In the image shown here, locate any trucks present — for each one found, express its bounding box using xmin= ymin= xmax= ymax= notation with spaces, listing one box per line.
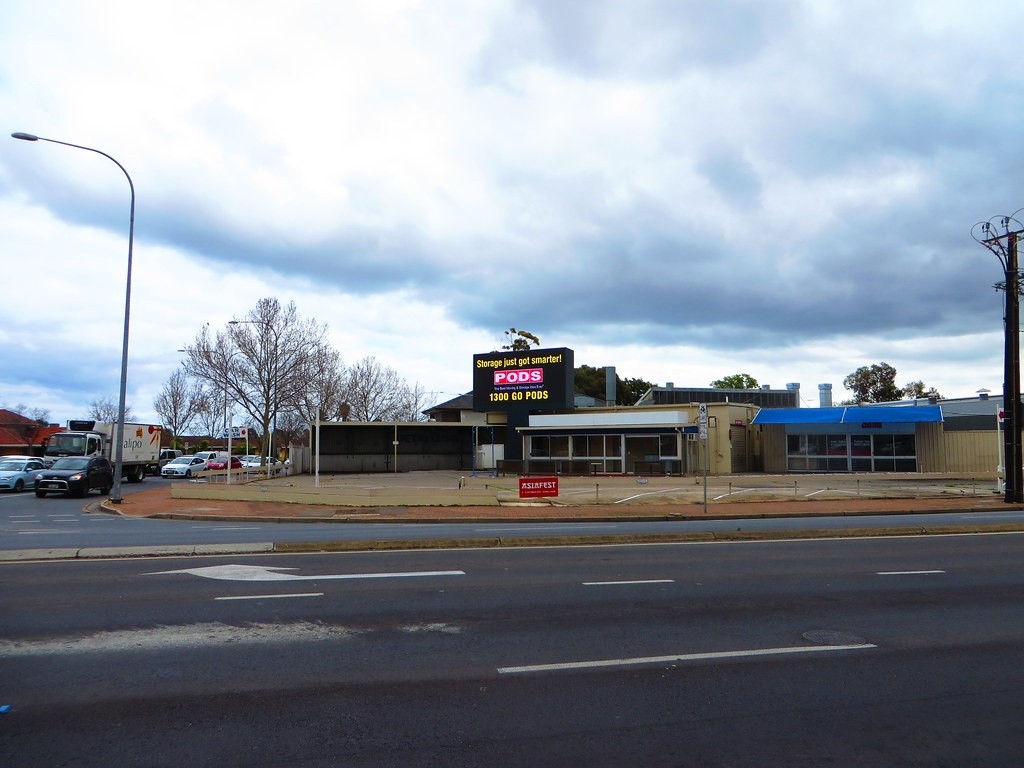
xmin=193 ymin=451 xmax=229 ymax=464
xmin=41 ymin=419 xmax=163 ymax=483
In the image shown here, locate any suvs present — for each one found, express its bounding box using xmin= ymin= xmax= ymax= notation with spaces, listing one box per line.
xmin=145 ymin=449 xmax=184 ymax=477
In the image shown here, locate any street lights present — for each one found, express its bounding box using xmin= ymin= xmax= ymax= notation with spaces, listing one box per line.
xmin=11 ymin=132 xmax=135 ymax=504
xmin=177 ymin=349 xmax=227 ymax=452
xmin=228 ymin=320 xmax=278 ymax=474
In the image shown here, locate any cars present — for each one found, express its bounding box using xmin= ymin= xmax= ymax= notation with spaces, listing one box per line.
xmin=161 ymin=456 xmax=208 ymax=478
xmin=207 ymin=456 xmax=242 ymax=475
xmin=242 ymin=456 xmax=283 ymax=476
xmin=34 ymin=456 xmax=115 ymax=500
xmin=0 ymin=461 xmax=51 ymax=494
xmin=238 ymin=455 xmax=258 ymax=466
xmin=0 ymin=455 xmax=44 ymax=467
